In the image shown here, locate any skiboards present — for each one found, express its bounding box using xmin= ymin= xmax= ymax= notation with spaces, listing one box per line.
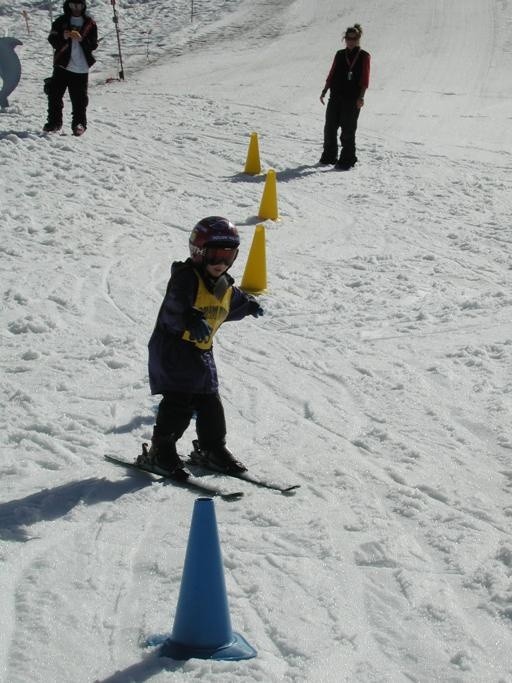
xmin=105 ymin=452 xmax=301 ymax=497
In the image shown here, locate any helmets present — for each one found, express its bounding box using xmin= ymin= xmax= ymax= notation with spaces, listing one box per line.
xmin=189 ymin=215 xmax=239 ymax=268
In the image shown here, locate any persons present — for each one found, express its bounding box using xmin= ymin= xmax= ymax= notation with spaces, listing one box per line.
xmin=142 ymin=216 xmax=265 ymax=478
xmin=319 ymin=24 xmax=371 ymax=170
xmin=43 ymin=0 xmax=98 ymax=137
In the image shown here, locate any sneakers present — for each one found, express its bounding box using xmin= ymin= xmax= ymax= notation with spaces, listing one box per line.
xmin=200 ymin=447 xmax=248 ymax=473
xmin=73 ymin=124 xmax=85 ymax=135
xmin=43 ymin=123 xmax=59 ymax=129
xmin=148 ymin=445 xmax=185 ymax=473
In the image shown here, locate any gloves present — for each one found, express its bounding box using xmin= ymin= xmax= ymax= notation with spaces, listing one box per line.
xmin=183 ymin=309 xmax=212 ymax=341
xmin=246 ymin=299 xmax=264 ymax=318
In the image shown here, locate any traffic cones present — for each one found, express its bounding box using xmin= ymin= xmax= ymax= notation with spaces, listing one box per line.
xmin=243 ymin=131 xmax=265 ymax=177
xmin=166 ymin=494 xmax=257 ymax=662
xmin=239 ymin=224 xmax=268 ymax=295
xmin=260 ymin=169 xmax=282 ymax=221
